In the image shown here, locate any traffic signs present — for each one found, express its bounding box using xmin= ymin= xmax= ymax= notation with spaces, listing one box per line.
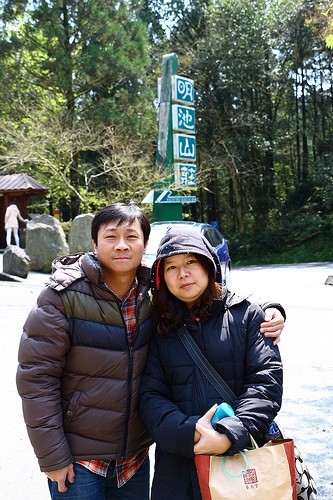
xmin=155 ymin=191 xmax=197 ymax=204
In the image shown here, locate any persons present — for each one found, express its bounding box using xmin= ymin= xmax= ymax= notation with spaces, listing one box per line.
xmin=16 ymin=202 xmax=286 ymax=500
xmin=4 ymin=200 xmax=27 ymax=246
xmin=141 ymin=226 xmax=283 ymax=500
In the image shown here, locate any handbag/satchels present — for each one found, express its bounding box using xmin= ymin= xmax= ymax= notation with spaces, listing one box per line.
xmin=291 ymin=446 xmax=317 ymax=500
xmin=194 ymin=434 xmax=298 ymax=500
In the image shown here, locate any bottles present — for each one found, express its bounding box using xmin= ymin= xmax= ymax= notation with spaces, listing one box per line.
xmin=211 ymin=402 xmax=235 ymax=429
